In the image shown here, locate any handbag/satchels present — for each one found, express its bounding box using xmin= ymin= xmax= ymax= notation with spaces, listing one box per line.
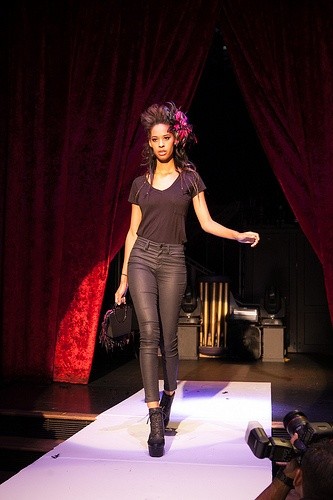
xmin=104 ymin=303 xmax=132 ymax=339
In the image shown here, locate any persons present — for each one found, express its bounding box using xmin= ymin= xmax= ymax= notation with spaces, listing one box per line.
xmin=115 ymin=104 xmax=260 ymax=456
xmin=255 ymin=432 xmax=333 ymax=500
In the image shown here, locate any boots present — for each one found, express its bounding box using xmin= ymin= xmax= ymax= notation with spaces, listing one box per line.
xmin=142 ymin=407 xmax=165 ymax=456
xmin=159 ymin=391 xmax=174 ymax=429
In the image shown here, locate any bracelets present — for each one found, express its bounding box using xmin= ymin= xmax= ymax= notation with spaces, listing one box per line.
xmin=122 ymin=274 xmax=127 ymax=276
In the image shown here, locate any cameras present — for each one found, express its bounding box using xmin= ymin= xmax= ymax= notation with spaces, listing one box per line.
xmin=244 ymin=409 xmax=333 ymax=466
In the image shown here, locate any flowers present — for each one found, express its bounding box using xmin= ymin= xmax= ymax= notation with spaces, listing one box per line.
xmin=174 ymin=108 xmax=198 ymax=146
